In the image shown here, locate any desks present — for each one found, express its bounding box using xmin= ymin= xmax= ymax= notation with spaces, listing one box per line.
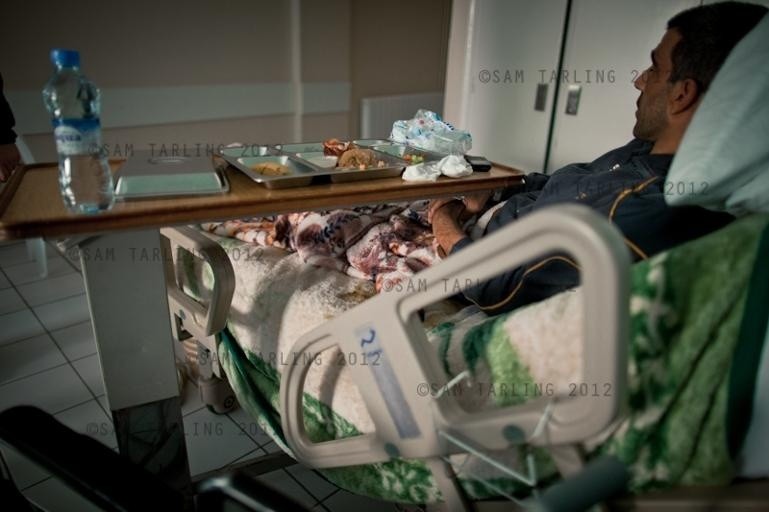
xmin=1 ymin=143 xmax=531 ymax=484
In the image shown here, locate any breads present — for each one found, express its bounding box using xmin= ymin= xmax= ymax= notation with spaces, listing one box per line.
xmin=338 ymin=149 xmax=385 ymax=170
xmin=323 ymin=138 xmax=361 ymax=156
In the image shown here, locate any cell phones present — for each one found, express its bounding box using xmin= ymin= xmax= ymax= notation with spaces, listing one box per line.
xmin=466 ymin=156 xmax=491 ymax=172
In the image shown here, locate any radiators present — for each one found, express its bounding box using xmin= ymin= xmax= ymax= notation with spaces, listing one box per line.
xmin=360 ymin=92 xmax=444 ymax=140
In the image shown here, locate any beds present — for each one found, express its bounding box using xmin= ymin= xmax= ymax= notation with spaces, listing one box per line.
xmin=159 ymin=176 xmax=769 ymax=512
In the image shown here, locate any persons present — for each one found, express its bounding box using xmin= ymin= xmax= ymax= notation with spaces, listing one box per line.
xmin=426 ymin=1 xmax=769 ymax=321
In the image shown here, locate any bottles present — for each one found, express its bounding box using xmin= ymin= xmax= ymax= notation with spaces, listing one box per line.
xmin=41 ymin=49 xmax=114 ymax=215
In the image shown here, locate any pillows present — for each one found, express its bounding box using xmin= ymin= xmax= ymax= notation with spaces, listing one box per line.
xmin=663 ymin=15 xmax=769 ymax=218
